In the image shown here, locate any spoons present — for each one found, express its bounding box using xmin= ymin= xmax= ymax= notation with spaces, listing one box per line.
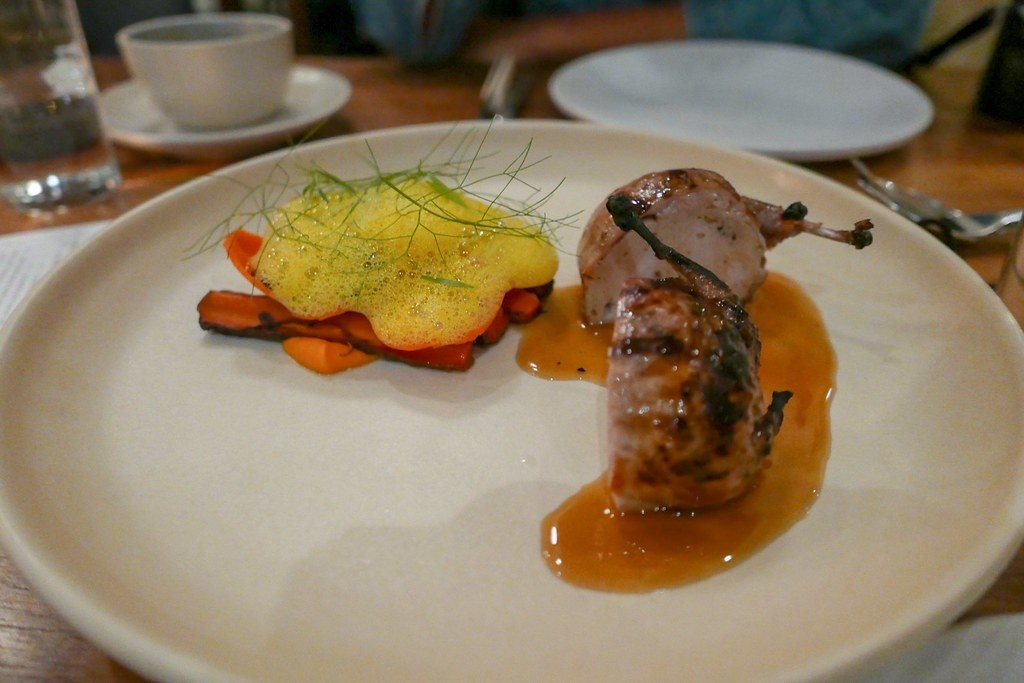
xmin=853 ymin=160 xmax=1024 ymax=243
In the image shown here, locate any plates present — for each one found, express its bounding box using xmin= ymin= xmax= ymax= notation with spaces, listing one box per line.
xmin=103 ymin=65 xmax=353 ymax=161
xmin=0 ymin=120 xmax=1024 ymax=683
xmin=549 ymin=41 xmax=936 ymax=162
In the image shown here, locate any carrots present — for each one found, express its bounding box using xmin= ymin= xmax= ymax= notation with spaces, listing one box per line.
xmin=195 ymin=231 xmax=542 ymax=370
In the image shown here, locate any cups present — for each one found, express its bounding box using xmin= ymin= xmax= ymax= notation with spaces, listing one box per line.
xmin=115 ymin=12 xmax=294 ymax=130
xmin=683 ymin=0 xmax=931 ymax=70
xmin=978 ymin=0 xmax=1024 ymax=125
xmin=0 ymin=0 xmax=121 ymax=215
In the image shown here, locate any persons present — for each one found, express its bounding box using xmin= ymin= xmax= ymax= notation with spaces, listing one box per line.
xmin=353 ymin=0 xmax=935 ymax=67
